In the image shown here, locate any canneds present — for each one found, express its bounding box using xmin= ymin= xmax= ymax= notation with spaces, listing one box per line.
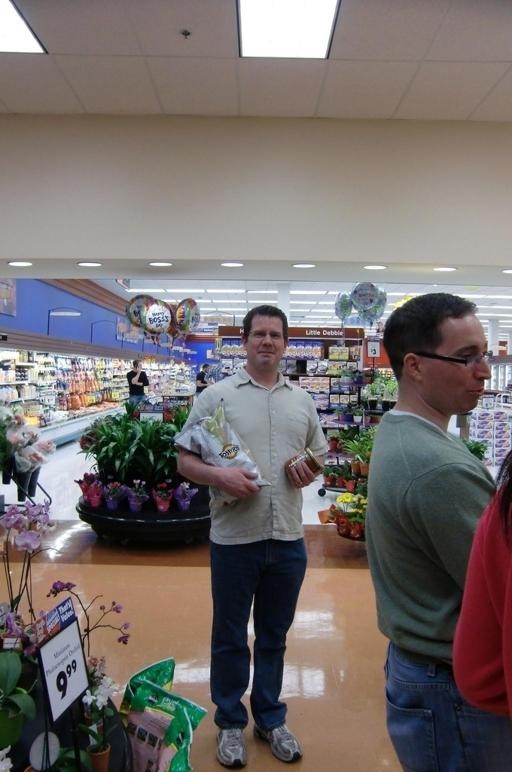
xmin=285 ymin=446 xmax=320 ymax=479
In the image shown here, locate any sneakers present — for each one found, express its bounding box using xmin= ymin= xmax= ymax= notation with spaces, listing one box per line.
xmin=254 ymin=723 xmax=303 ymax=762
xmin=216 ymin=728 xmax=246 ymax=767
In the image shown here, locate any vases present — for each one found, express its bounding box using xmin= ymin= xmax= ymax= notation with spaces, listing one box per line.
xmin=80 ymin=485 xmax=192 ymax=511
xmin=336 ymin=516 xmax=364 ymax=538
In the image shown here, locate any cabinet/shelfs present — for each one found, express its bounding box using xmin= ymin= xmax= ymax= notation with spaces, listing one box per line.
xmin=216 ymin=336 xmax=364 ymax=412
xmin=0 ymin=346 xmax=198 ymax=453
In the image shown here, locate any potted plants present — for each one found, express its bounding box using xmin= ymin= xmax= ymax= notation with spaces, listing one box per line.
xmin=80 ymin=685 xmax=111 ymax=772
xmin=322 ymin=402 xmax=379 ymax=493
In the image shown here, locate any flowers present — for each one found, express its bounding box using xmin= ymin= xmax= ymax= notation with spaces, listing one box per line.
xmin=78 ymin=474 xmax=199 ymax=500
xmin=336 ymin=492 xmax=367 ymax=523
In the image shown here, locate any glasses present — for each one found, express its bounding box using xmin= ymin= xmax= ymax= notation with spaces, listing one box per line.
xmin=414 ymin=350 xmax=493 ymax=367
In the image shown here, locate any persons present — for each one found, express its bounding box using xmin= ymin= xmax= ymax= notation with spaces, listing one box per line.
xmin=173 ymin=304 xmax=332 ymax=769
xmin=195 ymin=363 xmax=210 ymax=397
xmin=450 ymin=449 xmax=512 ymax=728
xmin=127 ymin=359 xmax=150 ymax=406
xmin=362 ymin=291 xmax=512 ymax=772
